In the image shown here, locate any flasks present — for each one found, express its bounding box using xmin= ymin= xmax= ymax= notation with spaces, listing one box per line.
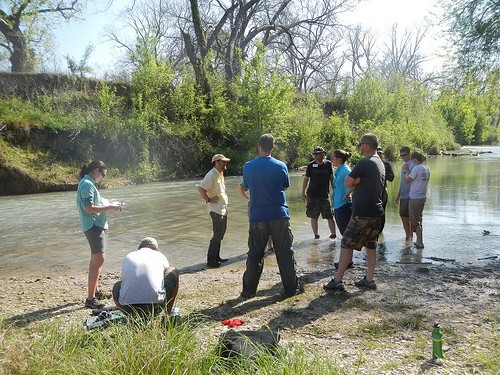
xmin=432 ymin=321 xmax=442 ymax=359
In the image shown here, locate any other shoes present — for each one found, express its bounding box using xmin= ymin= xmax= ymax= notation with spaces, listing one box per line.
xmin=244 ymin=291 xmax=254 ymax=298
xmin=218 ymin=259 xmax=228 ymax=262
xmin=315 ymin=235 xmax=319 ymax=238
xmin=330 ymin=235 xmax=336 ymax=238
xmin=208 ymin=261 xmax=221 ymax=266
xmin=334 ymin=261 xmax=353 ymax=267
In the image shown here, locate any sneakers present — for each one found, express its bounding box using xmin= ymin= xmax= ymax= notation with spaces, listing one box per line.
xmin=323 ymin=279 xmax=343 ymax=291
xmin=358 ymin=276 xmax=376 ymax=288
xmin=85 ymin=298 xmax=105 ymax=309
xmin=95 ymin=291 xmax=112 ymax=299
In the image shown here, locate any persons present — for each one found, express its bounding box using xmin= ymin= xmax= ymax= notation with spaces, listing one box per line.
xmin=395 ymin=146 xmax=413 ymax=241
xmin=241 ymin=133 xmax=305 ymax=299
xmin=238 ymin=181 xmax=274 ymax=255
xmin=323 ymin=132 xmax=386 ymax=291
xmin=403 ymin=151 xmax=430 ymax=249
xmin=376 ymin=146 xmax=394 ymax=243
xmin=76 ymin=160 xmax=124 ymax=308
xmin=302 ymin=146 xmax=336 ymax=239
xmin=198 ymin=154 xmax=230 ymax=268
xmin=332 ymin=149 xmax=354 ymax=268
xmin=113 ymin=236 xmax=181 ymax=324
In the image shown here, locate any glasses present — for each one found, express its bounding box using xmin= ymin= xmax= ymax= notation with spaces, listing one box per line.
xmin=400 ymin=153 xmax=409 ymax=157
xmin=359 ymin=142 xmax=370 ymax=146
xmin=100 ymin=171 xmax=105 ymax=178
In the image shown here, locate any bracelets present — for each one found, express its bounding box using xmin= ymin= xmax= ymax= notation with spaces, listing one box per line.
xmin=345 ymin=193 xmax=349 ymax=196
xmin=404 ymin=171 xmax=407 ymax=174
xmin=206 ymin=198 xmax=210 ymax=204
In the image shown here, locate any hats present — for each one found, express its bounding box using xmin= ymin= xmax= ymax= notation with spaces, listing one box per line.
xmin=314 ymin=148 xmax=325 ymax=153
xmin=212 ymin=154 xmax=230 ymax=162
xmin=377 ymin=146 xmax=382 ymax=153
